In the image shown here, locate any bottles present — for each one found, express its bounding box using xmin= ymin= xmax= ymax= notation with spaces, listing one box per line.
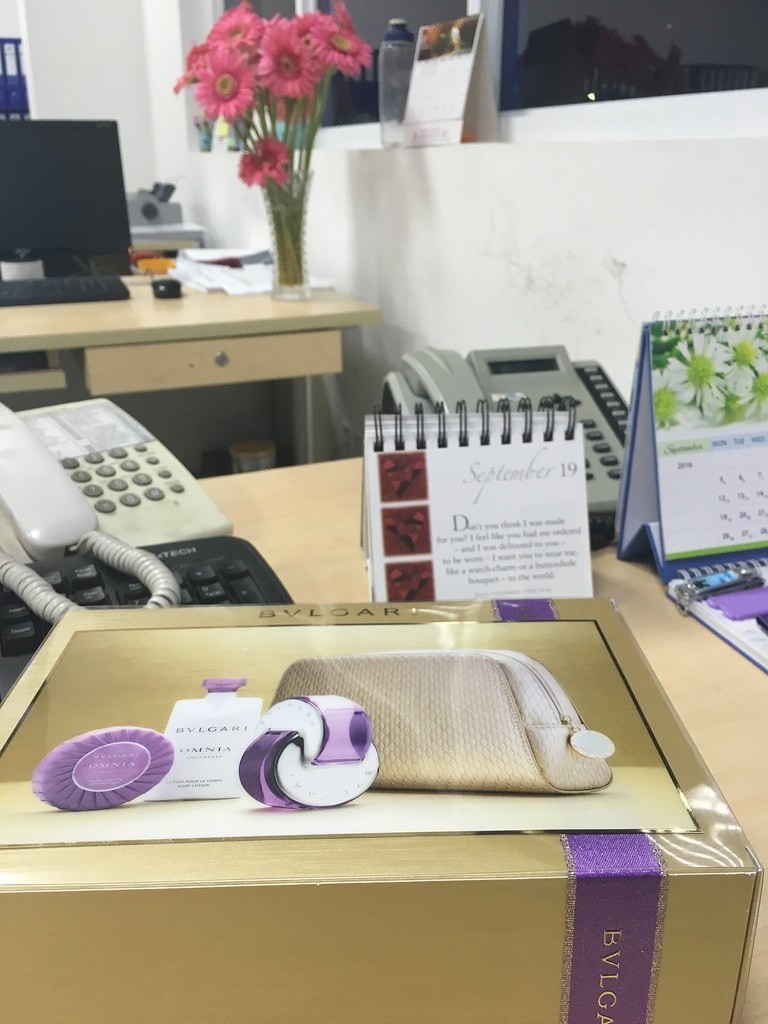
xmin=143 ymin=678 xmax=265 ymax=800
xmin=378 ymin=18 xmax=416 ymax=148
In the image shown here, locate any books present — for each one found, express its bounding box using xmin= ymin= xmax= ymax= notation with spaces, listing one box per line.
xmin=163 ymin=243 xmax=276 ymax=293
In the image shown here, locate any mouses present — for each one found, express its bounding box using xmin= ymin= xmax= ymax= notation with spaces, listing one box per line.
xmin=153 ymin=279 xmax=180 ymax=298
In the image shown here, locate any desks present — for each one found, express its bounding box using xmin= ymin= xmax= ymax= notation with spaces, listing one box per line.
xmin=0 ymin=274 xmax=384 ymax=464
xmin=192 ymin=459 xmax=768 ymax=1024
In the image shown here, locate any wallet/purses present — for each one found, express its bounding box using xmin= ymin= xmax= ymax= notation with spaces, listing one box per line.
xmin=268 ymin=650 xmax=615 ymax=793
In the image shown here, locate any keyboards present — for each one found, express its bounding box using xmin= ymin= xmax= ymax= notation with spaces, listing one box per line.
xmin=0 ymin=273 xmax=131 ymax=307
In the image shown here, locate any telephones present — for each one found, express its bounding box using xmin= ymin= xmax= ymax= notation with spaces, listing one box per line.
xmin=0 ymin=397 xmax=235 ymax=565
xmin=379 ymin=344 xmax=636 ymax=545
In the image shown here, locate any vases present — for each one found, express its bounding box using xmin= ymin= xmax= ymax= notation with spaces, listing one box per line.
xmin=258 ymin=171 xmax=313 ymax=301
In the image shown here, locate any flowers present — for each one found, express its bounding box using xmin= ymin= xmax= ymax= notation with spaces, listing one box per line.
xmin=174 ymin=0 xmax=372 ymax=285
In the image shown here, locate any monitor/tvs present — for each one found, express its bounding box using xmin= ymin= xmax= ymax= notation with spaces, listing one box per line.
xmin=0 ymin=118 xmax=132 ymax=279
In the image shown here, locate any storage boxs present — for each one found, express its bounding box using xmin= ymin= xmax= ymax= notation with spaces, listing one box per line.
xmin=0 ymin=597 xmax=764 ymax=1024
